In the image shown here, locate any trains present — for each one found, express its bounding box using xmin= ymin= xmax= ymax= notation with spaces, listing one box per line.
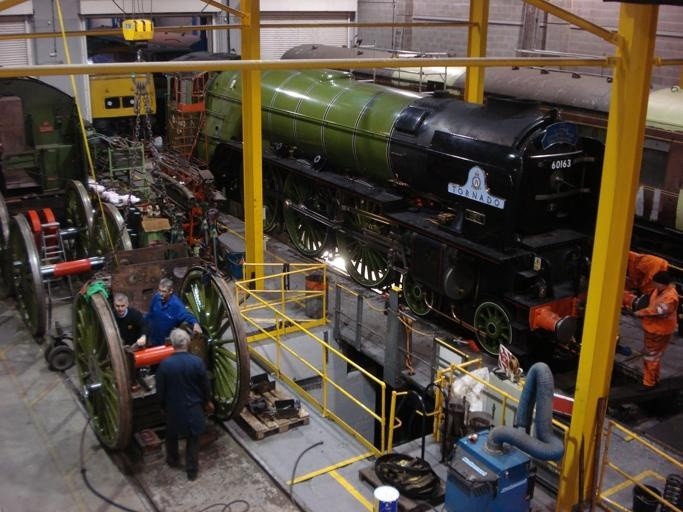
xmin=188 ymin=62 xmax=647 ymax=370
xmin=84 ymin=30 xmax=161 ymax=137
xmin=284 ymin=43 xmax=681 ymax=289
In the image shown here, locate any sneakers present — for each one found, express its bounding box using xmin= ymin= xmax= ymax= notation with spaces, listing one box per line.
xmin=187 ymin=467 xmax=197 ymax=480
xmin=637 ymin=385 xmax=657 ymax=392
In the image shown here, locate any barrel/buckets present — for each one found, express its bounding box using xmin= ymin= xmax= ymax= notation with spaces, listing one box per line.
xmin=374 ymin=485 xmax=401 ymax=511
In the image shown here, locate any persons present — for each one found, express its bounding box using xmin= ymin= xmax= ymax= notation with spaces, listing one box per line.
xmin=144 ymin=278 xmax=202 ymax=374
xmin=634 ymin=271 xmax=679 ymax=391
xmin=157 ymin=328 xmax=211 ymax=479
xmin=113 ymin=292 xmax=149 ymax=387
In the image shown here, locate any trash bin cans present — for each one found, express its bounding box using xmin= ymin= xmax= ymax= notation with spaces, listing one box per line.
xmin=633 ymin=483 xmax=661 ymax=511
xmin=306 ymin=275 xmax=328 ymax=319
